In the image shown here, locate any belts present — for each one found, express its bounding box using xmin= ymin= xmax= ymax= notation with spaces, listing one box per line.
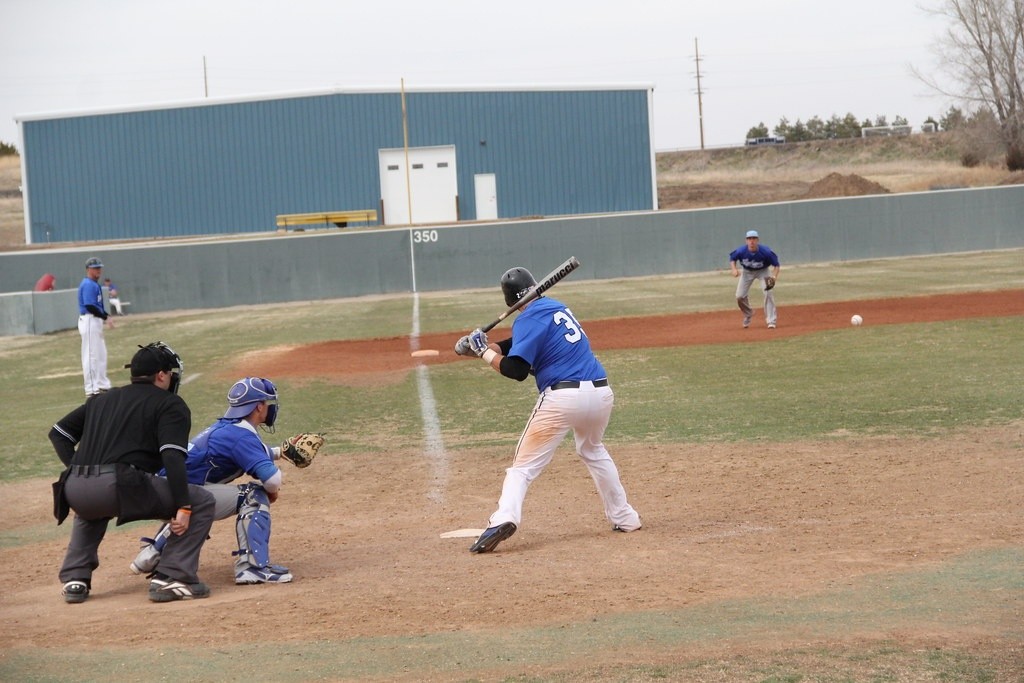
xmin=71 ymin=464 xmax=114 ymax=475
xmin=551 ymin=379 xmax=608 ymax=390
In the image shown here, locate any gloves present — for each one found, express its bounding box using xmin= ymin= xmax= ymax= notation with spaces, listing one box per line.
xmin=455 ymin=335 xmax=478 ymax=356
xmin=467 ymin=328 xmax=488 ymax=356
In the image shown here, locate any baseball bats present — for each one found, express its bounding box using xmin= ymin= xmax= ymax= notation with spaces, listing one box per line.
xmin=454 ymin=256 xmax=580 ymax=356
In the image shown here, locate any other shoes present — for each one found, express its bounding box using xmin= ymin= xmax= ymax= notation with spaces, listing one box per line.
xmin=743 ymin=317 xmax=750 ymax=327
xmin=768 ymin=324 xmax=776 ymax=328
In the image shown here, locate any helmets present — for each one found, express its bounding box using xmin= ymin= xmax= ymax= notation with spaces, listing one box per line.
xmin=225 ymin=377 xmax=279 ymax=434
xmin=500 ymin=267 xmax=538 ymax=307
xmin=131 ymin=341 xmax=183 ymax=392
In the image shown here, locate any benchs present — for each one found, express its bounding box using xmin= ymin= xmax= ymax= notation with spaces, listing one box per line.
xmin=110 ymin=301 xmax=130 ymax=312
xmin=275 ymin=209 xmax=377 ymax=232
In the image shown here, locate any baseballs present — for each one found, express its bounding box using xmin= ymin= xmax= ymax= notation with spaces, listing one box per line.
xmin=850 ymin=315 xmax=862 ymax=325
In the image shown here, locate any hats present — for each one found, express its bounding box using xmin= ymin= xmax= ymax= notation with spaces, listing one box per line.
xmin=746 ymin=231 xmax=759 ymax=238
xmin=85 ymin=257 xmax=104 ymax=268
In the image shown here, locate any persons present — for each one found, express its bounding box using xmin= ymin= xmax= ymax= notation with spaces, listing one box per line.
xmin=103 ymin=278 xmax=124 ymax=315
xmin=454 ymin=266 xmax=641 ymax=554
xmin=129 ymin=376 xmax=326 ymax=585
xmin=78 ymin=256 xmax=112 ymax=399
xmin=48 ymin=341 xmax=216 ymax=604
xmin=729 ymin=230 xmax=781 ymax=328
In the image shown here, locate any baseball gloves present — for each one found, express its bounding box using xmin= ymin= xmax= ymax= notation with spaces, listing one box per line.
xmin=281 ymin=431 xmax=325 ymax=468
xmin=764 ymin=275 xmax=775 ymax=290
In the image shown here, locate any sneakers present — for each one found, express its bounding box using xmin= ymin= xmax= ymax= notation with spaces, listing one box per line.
xmin=613 ymin=525 xmax=641 ymax=532
xmin=470 ymin=521 xmax=516 ymax=553
xmin=148 ymin=570 xmax=210 ymax=602
xmin=62 ymin=581 xmax=89 ymax=603
xmin=130 ymin=562 xmax=142 ymax=575
xmin=235 ymin=565 xmax=293 ymax=583
